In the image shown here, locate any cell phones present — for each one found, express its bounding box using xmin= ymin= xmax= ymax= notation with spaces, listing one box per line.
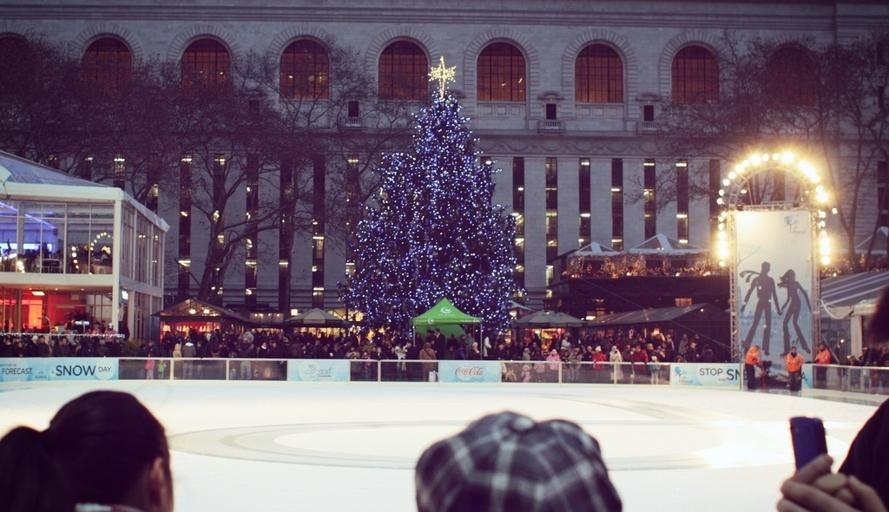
xmin=790 ymin=416 xmax=827 ymax=470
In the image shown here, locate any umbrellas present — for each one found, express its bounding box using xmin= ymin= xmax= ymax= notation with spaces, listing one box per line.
xmin=283 ymin=306 xmax=353 ymax=335
xmin=528 ymin=311 xmax=588 ymax=340
xmin=514 ymin=309 xmax=551 ymax=338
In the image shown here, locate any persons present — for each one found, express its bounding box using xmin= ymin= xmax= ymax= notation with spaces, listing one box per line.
xmin=0 ymin=391 xmax=174 ymax=512
xmin=777 ymin=270 xmax=811 ymax=356
xmin=848 ymin=344 xmax=889 ymax=387
xmin=775 ymin=283 xmax=889 ymax=512
xmin=0 ymin=239 xmax=110 ymax=273
xmin=739 ymin=261 xmax=780 ymax=355
xmin=0 ymin=328 xmax=689 ymax=384
xmin=785 ymin=346 xmax=805 ymax=391
xmin=745 ymin=345 xmax=760 ymax=389
xmin=815 ymin=343 xmax=831 ymax=380
